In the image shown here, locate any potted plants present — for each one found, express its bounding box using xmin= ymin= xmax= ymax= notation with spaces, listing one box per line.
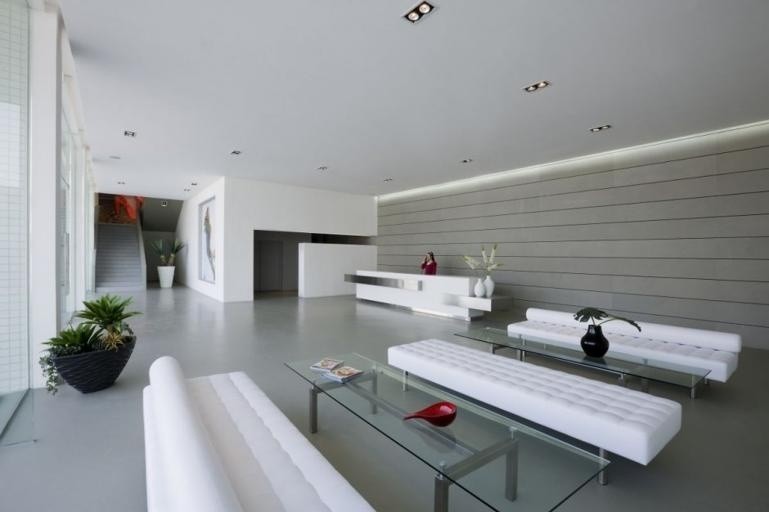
xmin=574 ymin=308 xmax=640 ymax=358
xmin=38 ymin=292 xmax=144 ymax=397
xmin=148 ymin=237 xmax=184 ymax=289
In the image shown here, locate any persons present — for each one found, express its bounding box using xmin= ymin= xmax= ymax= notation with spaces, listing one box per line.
xmin=419 ymin=251 xmax=438 ymax=275
xmin=104 ymin=195 xmax=125 ymax=223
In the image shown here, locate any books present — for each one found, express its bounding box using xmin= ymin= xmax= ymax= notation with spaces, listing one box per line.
xmin=310 ymin=358 xmax=344 ymax=372
xmin=323 ymin=366 xmax=363 ymax=383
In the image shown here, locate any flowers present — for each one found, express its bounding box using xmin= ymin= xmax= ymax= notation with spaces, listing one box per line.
xmin=461 ymin=243 xmax=504 ymax=275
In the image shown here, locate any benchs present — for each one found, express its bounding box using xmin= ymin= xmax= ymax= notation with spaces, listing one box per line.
xmin=507 ymin=307 xmax=742 ymax=384
xmin=142 ymin=356 xmax=379 ymax=512
xmin=387 ymin=340 xmax=683 ymax=484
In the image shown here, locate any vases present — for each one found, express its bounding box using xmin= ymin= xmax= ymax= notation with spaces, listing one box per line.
xmin=484 ymin=276 xmax=495 ymax=297
xmin=474 ymin=278 xmax=483 ymax=297
